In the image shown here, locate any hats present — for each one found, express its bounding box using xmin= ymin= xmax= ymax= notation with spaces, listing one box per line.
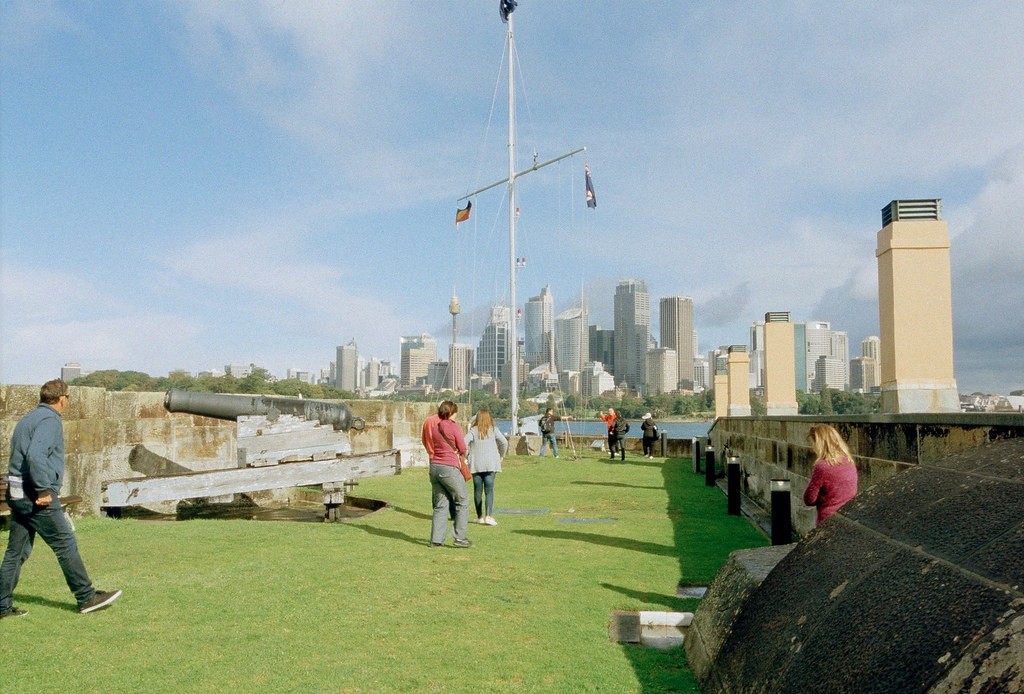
xmin=437 ymin=399 xmax=450 ymax=409
xmin=642 ymin=412 xmax=651 ymax=420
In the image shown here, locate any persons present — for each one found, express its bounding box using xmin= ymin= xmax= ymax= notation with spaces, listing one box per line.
xmin=428 ymin=401 xmax=473 ymax=547
xmin=609 ymin=410 xmax=630 ymax=461
xmin=422 ymin=400 xmax=469 ymax=521
xmin=803 ymin=423 xmax=857 ymax=527
xmin=599 ymin=408 xmax=620 ymax=456
xmin=0 ymin=379 xmax=122 ymax=619
xmin=464 ymin=408 xmax=508 ymax=526
xmin=641 ymin=413 xmax=658 ymax=459
xmin=539 ymin=407 xmax=573 ymax=458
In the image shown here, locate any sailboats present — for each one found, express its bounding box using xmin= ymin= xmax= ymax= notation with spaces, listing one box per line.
xmin=452 ymin=0 xmax=589 ymax=436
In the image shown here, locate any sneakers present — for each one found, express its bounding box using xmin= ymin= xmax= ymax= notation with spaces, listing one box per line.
xmin=0 ymin=610 xmax=29 ymax=617
xmin=78 ymin=589 xmax=123 ymax=613
xmin=473 ymin=516 xmax=497 ymax=526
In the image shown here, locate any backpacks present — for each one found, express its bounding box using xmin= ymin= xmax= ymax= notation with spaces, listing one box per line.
xmin=540 ymin=416 xmax=553 ymax=433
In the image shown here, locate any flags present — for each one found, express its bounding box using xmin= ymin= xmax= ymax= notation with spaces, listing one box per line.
xmin=455 ymin=201 xmax=471 ymax=223
xmin=585 ymin=161 xmax=597 ymax=209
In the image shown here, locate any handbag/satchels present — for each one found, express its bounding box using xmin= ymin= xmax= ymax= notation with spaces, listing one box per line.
xmin=653 ymin=427 xmax=660 ymax=440
xmin=459 ymin=457 xmax=473 ymax=481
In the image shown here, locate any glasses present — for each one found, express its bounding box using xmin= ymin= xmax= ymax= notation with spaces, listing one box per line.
xmin=60 ymin=395 xmax=70 ymax=399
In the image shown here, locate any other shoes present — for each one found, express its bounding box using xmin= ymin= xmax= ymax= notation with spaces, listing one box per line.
xmin=649 ymin=456 xmax=654 ymax=459
xmin=556 ymin=456 xmax=559 ymax=458
xmin=435 ymin=543 xmax=442 ymax=546
xmin=622 ymin=461 xmax=624 ymax=462
xmin=453 ymin=538 xmax=471 ymax=548
xmin=609 ymin=458 xmax=614 ymax=461
xmin=644 ymin=455 xmax=649 ymax=458
